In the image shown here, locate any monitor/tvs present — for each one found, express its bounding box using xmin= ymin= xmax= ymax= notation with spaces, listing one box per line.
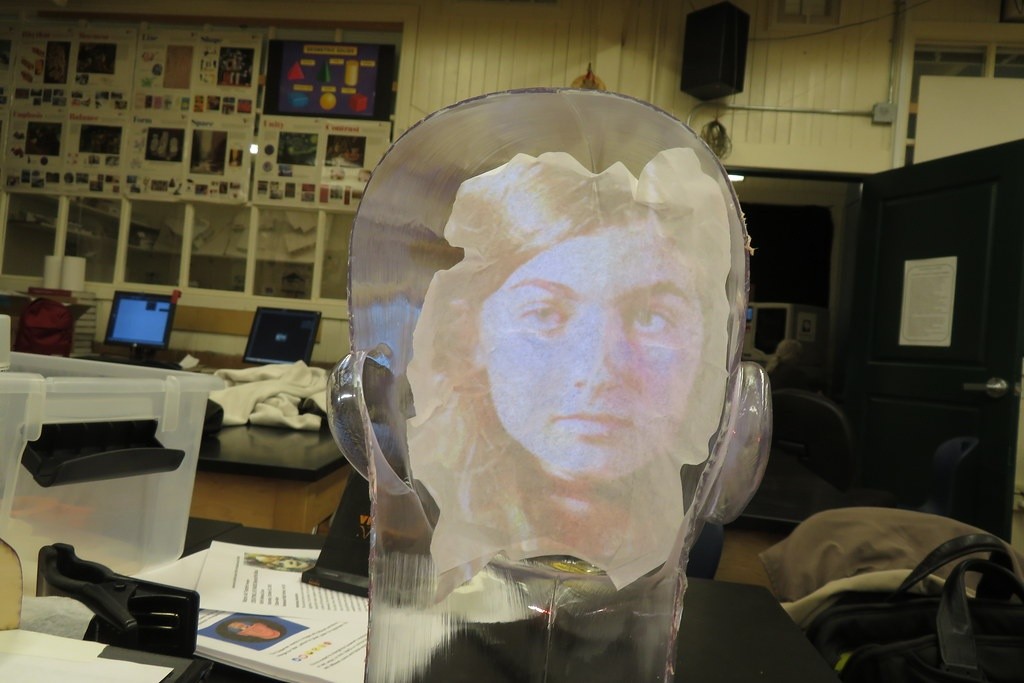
xmin=242 ymin=306 xmax=322 ymax=368
xmin=102 ymin=290 xmax=176 ymax=365
xmin=262 ymin=40 xmax=396 ymax=122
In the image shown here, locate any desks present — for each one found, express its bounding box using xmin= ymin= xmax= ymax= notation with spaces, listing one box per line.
xmin=182 ymin=516 xmax=843 ymax=683
xmin=187 ymin=422 xmax=354 ymax=553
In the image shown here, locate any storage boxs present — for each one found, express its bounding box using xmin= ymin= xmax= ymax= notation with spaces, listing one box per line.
xmin=0 ymin=351 xmax=225 ymax=630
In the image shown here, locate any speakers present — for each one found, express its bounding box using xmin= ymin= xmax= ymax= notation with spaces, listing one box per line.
xmin=679 ymin=0 xmax=750 ymax=101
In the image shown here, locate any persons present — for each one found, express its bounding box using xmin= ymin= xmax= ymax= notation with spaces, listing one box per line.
xmin=412 ymin=144 xmax=728 ymax=569
xmin=760 ymin=340 xmax=818 ymax=394
xmin=328 ymin=85 xmax=773 ymax=682
